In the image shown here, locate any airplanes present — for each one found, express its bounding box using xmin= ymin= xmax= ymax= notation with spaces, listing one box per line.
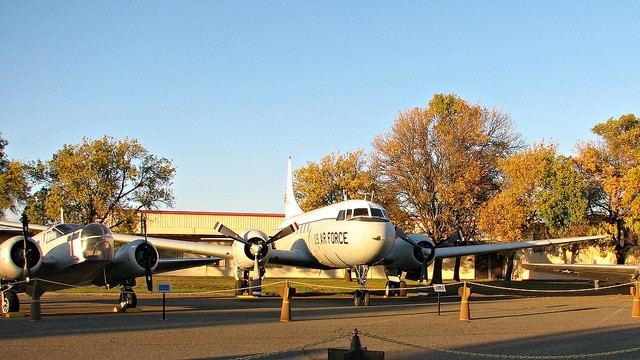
xmin=5 ymin=157 xmax=610 ymax=306
xmin=0 ymin=211 xmax=223 ymax=313
